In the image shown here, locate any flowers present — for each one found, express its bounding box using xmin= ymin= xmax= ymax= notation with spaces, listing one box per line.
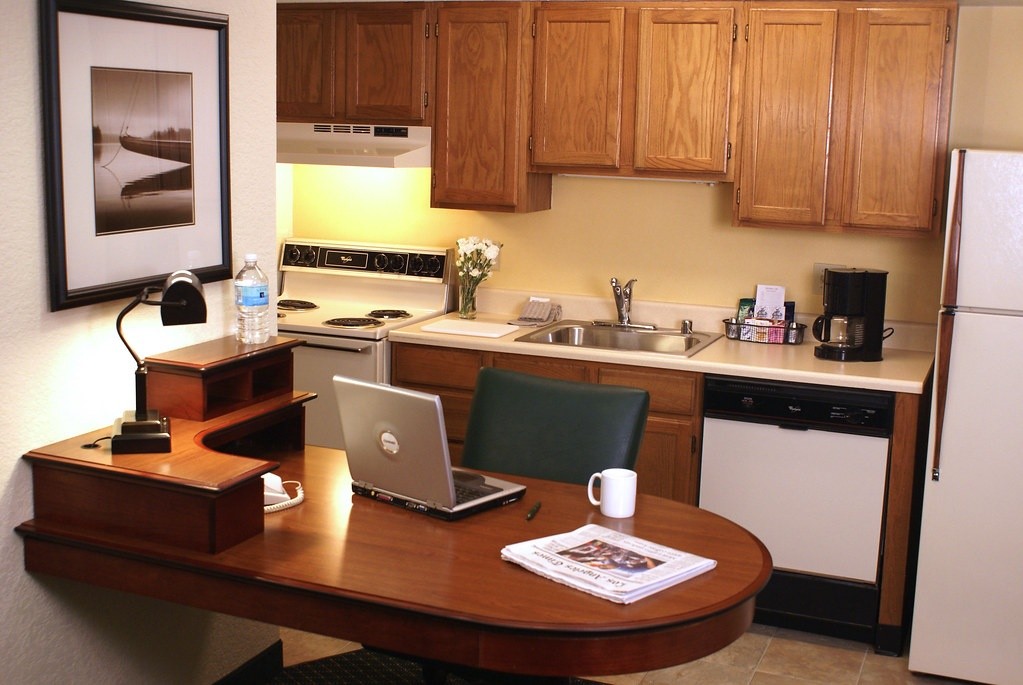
xmin=456 ymin=237 xmax=503 ymax=286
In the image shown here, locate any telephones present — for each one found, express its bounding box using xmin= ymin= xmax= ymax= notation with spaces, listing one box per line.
xmin=261 ymin=472 xmax=291 ymax=505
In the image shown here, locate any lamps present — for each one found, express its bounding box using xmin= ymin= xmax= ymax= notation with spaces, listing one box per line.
xmin=111 ymin=270 xmax=208 ymax=454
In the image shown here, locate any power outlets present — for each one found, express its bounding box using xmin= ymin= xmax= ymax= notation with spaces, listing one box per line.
xmin=811 ymin=262 xmax=847 ymax=295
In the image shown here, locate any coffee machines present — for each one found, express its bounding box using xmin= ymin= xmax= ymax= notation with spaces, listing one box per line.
xmin=812 ymin=267 xmax=890 ymax=363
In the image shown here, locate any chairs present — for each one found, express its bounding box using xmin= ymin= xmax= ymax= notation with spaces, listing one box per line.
xmin=362 ymin=366 xmax=650 ymax=685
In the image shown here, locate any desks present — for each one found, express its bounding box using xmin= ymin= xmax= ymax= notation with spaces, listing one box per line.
xmin=13 ymin=335 xmax=774 ymax=685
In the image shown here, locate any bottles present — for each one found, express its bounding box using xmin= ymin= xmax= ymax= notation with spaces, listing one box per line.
xmin=234 ymin=253 xmax=271 ymax=344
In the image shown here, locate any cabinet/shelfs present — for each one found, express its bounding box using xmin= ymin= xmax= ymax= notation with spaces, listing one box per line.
xmin=276 ymin=0 xmax=435 ymax=127
xmin=594 ymin=361 xmax=705 ymax=505
xmin=526 ymin=0 xmax=742 ymax=183
xmin=732 ymin=0 xmax=960 ymax=241
xmin=390 ymin=341 xmax=488 ymax=466
xmin=429 ymin=0 xmax=553 ymax=214
xmin=486 ymin=351 xmax=596 ymax=384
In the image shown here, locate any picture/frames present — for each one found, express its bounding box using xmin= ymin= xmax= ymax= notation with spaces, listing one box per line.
xmin=38 ymin=0 xmax=233 ymax=312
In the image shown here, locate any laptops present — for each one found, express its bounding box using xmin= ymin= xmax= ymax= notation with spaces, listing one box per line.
xmin=333 ymin=373 xmax=526 ymax=520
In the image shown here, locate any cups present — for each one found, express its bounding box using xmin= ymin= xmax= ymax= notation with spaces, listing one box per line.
xmin=587 ymin=468 xmax=639 ymax=518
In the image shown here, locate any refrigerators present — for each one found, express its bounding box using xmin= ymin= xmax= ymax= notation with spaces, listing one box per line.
xmin=909 ymin=143 xmax=1023 ymax=685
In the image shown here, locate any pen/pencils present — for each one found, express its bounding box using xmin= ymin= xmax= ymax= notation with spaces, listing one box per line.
xmin=527 ymin=501 xmax=542 ymax=520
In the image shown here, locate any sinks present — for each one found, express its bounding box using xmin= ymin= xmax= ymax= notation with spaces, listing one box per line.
xmin=514 ymin=319 xmax=726 ymax=359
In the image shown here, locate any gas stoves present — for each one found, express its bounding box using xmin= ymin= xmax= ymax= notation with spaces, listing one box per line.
xmin=271 ymin=239 xmax=455 ymax=340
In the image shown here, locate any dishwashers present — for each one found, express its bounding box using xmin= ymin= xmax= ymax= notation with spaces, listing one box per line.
xmin=276 ymin=329 xmax=391 ymax=452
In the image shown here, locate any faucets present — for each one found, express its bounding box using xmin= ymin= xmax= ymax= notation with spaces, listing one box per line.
xmin=610 ymin=277 xmax=638 ymax=323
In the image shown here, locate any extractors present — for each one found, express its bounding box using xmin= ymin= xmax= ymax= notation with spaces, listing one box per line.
xmin=274 ymin=120 xmax=432 ymax=167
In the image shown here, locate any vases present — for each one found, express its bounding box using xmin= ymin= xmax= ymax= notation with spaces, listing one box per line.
xmin=458 ymin=285 xmax=479 ymax=320
